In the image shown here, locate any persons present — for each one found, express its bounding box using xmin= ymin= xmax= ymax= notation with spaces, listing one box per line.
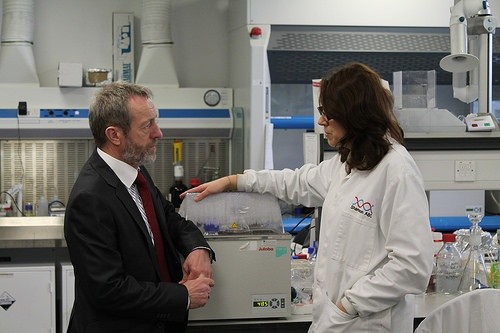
xmin=180 ymin=62 xmax=434 ymax=333
xmin=64 ymin=80 xmax=216 ymax=333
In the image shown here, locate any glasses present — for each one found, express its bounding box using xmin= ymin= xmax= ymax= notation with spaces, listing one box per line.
xmin=317 ymin=105 xmax=332 ymax=121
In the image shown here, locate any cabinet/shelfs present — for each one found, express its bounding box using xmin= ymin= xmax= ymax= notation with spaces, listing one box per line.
xmin=0 ymin=240 xmax=72 ymax=333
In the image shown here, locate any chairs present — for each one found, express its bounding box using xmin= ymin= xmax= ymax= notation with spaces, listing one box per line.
xmin=412 ymin=286 xmax=500 ymax=333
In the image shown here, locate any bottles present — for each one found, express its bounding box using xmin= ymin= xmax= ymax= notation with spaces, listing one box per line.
xmin=38 ymin=196 xmax=48 ymax=216
xmin=25 ymin=202 xmax=32 ymax=217
xmin=190 ymin=178 xmax=201 ymax=189
xmin=168 ymin=165 xmax=189 ymax=208
xmin=491 ymin=229 xmax=500 ymax=289
xmin=457 ymin=207 xmax=492 ymax=296
xmin=295 ymin=254 xmax=310 ymax=281
xmin=291 ymin=256 xmax=301 ymax=283
xmin=307 ymin=246 xmax=317 ymax=282
xmin=52 ymin=195 xmax=62 ymax=208
xmin=436 ymin=234 xmax=464 ymax=295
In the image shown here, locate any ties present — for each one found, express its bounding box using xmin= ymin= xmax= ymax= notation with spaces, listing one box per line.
xmin=135 ymin=171 xmax=171 ymax=283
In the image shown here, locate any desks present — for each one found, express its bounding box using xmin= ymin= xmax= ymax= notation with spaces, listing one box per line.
xmin=188 ymin=281 xmax=469 ymax=333
